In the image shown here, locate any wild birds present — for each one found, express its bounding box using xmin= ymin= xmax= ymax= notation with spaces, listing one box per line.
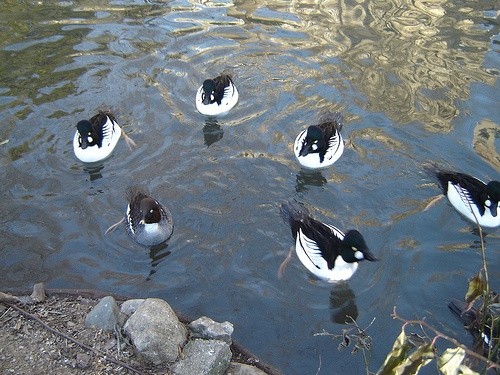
xmin=294 ymin=110 xmax=344 ymax=168
xmin=423 ymin=161 xmax=500 ymax=228
xmin=122 ymin=183 xmax=175 ymax=247
xmin=273 ymin=196 xmax=380 ymax=282
xmin=195 ymin=68 xmax=238 ymax=115
xmin=73 ymin=103 xmax=122 ymax=163
xmin=448 ymin=289 xmax=500 ymax=337
